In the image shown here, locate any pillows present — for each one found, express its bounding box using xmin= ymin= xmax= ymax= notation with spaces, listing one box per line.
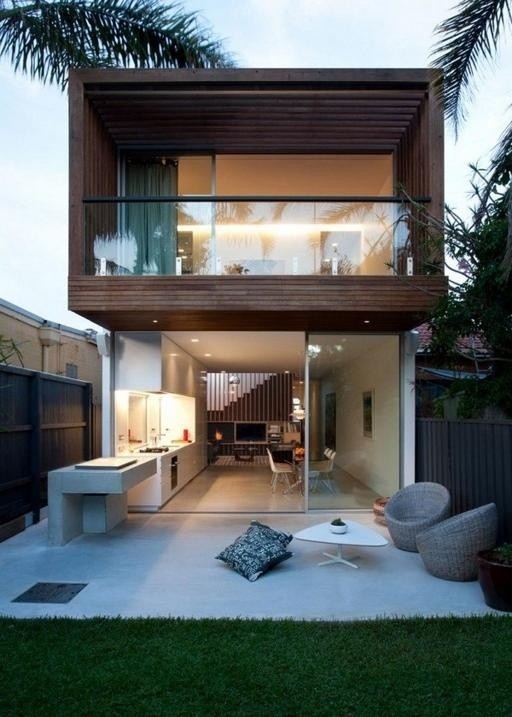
xmin=214 ymin=519 xmax=296 ymax=582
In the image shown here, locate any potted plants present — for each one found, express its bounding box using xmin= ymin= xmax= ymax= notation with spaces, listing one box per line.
xmin=476 ymin=542 xmax=512 ymax=612
xmin=329 ymin=517 xmax=348 ymax=534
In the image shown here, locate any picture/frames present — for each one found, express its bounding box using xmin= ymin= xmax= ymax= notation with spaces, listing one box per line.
xmin=361 ymin=388 xmax=375 ymax=440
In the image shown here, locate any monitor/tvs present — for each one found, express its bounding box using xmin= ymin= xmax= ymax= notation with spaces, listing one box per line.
xmin=234 ymin=421 xmax=268 ymax=445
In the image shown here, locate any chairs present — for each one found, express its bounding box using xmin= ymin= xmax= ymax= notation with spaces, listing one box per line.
xmin=384 ymin=482 xmax=498 ymax=581
xmin=266 ymin=446 xmax=336 ymax=497
